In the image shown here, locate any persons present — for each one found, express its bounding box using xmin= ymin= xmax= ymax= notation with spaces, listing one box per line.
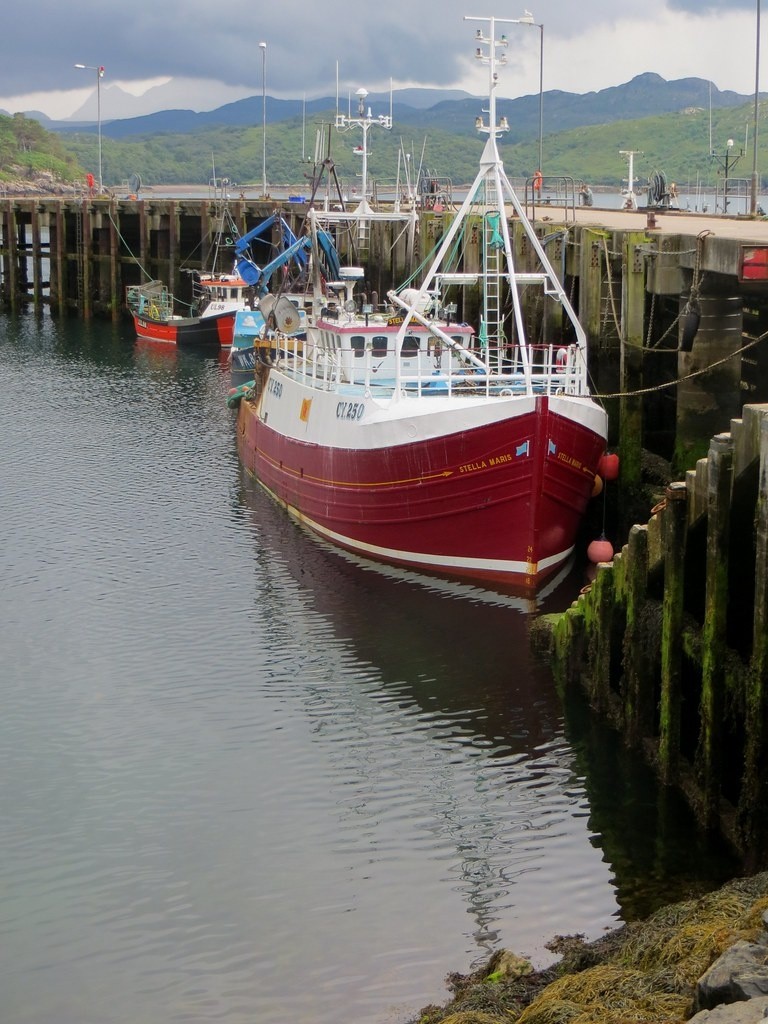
xmin=581 ymin=184 xmax=593 ymax=206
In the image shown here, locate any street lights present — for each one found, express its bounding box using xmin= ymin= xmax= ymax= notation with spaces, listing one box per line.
xmin=73 ymin=64 xmax=104 ymax=193
xmin=258 ymin=42 xmax=268 ymax=201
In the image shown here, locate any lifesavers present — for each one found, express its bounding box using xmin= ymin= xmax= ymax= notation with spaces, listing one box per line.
xmin=555 ymin=348 xmax=568 ymax=373
xmin=533 ymin=171 xmax=543 ymax=190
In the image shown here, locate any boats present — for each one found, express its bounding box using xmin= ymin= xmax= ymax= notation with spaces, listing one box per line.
xmin=226 ymin=9 xmax=611 ymax=600
xmin=124 ymin=178 xmax=259 ymax=348
